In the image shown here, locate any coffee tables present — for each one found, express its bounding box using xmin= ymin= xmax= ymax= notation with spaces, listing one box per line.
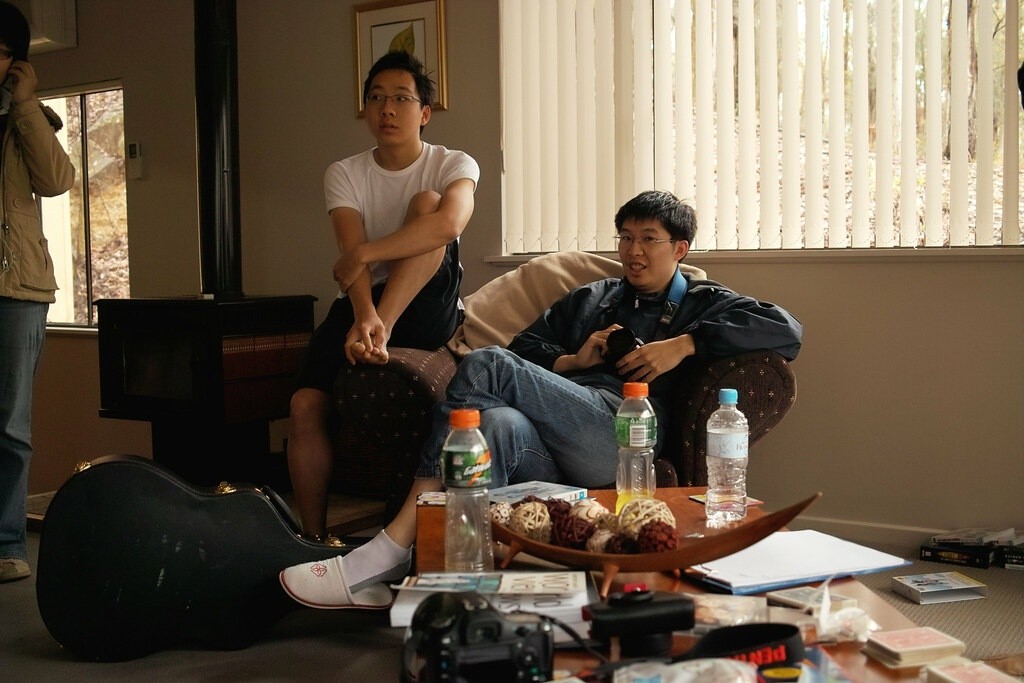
xmin=415 ymin=487 xmax=918 ymax=683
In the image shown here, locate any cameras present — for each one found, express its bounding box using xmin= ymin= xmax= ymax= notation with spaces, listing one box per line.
xmin=399 ymin=589 xmax=556 ymax=683
xmin=598 ymin=327 xmax=649 ymax=382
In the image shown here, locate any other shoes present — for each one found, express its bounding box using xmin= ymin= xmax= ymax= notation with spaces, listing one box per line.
xmin=0 ymin=557 xmax=31 ymax=579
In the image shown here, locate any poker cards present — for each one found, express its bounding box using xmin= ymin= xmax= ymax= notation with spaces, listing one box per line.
xmin=859 ymin=626 xmax=969 ymax=670
xmin=924 ymin=661 xmax=1022 ymax=683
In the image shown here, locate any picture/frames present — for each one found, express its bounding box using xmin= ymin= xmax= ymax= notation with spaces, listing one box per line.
xmin=351 ymin=0 xmax=448 ymax=121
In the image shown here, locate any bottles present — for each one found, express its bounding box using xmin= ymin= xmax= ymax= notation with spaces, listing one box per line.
xmin=440 ymin=408 xmax=495 ymax=572
xmin=705 ymin=388 xmax=749 ymax=530
xmin=615 ymin=382 xmax=658 ymax=515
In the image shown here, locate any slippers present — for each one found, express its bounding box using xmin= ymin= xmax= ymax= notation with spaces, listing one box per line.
xmin=279 ymin=555 xmax=394 ymax=609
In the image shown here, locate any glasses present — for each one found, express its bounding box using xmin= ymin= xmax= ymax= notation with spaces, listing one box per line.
xmin=366 ymin=92 xmax=423 ymax=105
xmin=0 ymin=49 xmax=12 ymax=59
xmin=615 ymin=231 xmax=675 ymax=249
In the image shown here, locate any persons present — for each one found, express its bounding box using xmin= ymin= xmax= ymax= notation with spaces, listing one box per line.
xmin=287 ymin=51 xmax=480 ymax=541
xmin=0 ymin=0 xmax=77 ymax=581
xmin=281 ymin=191 xmax=802 ymax=610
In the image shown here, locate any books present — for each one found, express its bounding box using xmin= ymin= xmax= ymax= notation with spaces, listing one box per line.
xmin=486 ymin=480 xmax=588 ymax=508
xmin=390 ymin=570 xmax=602 ymax=643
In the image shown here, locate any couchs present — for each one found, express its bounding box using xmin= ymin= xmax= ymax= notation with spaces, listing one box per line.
xmin=336 ymin=250 xmax=799 ymax=531
